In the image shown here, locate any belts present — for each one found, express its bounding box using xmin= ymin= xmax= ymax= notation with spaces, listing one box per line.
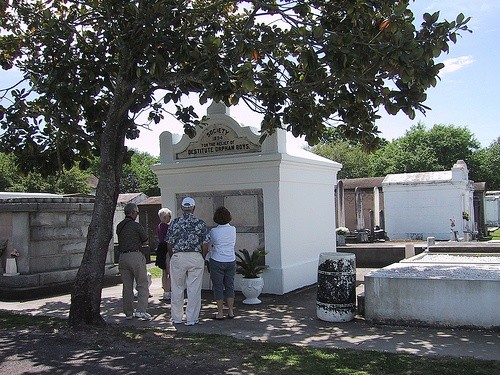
xmin=123 ymin=250 xmax=136 ymax=254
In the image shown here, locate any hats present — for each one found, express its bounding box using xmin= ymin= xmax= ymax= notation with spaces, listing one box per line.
xmin=181 ymin=197 xmax=196 ymax=208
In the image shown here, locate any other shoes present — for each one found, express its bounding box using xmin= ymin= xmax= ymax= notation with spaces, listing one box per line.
xmin=126 ymin=313 xmax=135 ymax=320
xmin=187 ymin=319 xmax=199 ymax=325
xmin=135 ymin=311 xmax=151 ymax=318
xmin=163 ymin=291 xmax=171 ymax=300
xmin=171 ymin=318 xmax=181 ymax=323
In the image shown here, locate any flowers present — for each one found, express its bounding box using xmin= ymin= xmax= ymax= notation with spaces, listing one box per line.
xmin=336 ymin=227 xmax=351 ymax=235
xmin=11 ymin=248 xmax=20 ymax=259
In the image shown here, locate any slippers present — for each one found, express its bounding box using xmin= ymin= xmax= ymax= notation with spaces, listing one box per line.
xmin=210 ymin=315 xmax=224 ymax=320
xmin=228 ymin=314 xmax=235 ymax=319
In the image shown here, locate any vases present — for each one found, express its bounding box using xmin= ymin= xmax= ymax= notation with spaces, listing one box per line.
xmin=6 ymin=258 xmax=17 ymax=274
xmin=337 ymin=234 xmax=346 ymax=246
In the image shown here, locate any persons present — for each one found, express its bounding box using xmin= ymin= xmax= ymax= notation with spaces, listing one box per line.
xmin=164 ymin=197 xmax=206 ymax=325
xmin=116 ymin=203 xmax=151 ymax=319
xmin=208 ymin=207 xmax=236 ymax=320
xmin=157 ymin=208 xmax=173 ymax=299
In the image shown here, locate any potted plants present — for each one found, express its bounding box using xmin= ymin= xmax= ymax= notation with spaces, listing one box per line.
xmin=235 ymin=246 xmax=270 ymax=305
